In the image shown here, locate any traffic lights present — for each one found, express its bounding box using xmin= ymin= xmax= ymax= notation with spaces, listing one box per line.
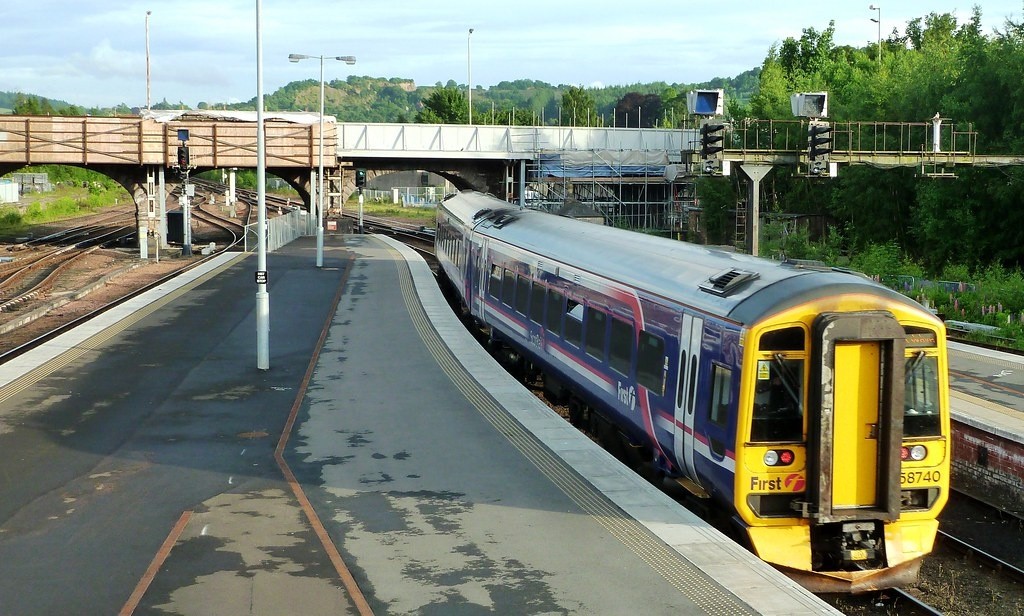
xmin=178 ymin=146 xmax=189 ymax=165
xmin=356 ymin=169 xmax=366 ymax=187
xmin=178 ymin=128 xmax=190 ymax=141
xmin=799 ymin=92 xmax=827 ymax=117
xmin=700 ymin=119 xmax=724 ymax=159
xmin=808 ymin=121 xmax=833 ymax=161
xmin=690 ymin=87 xmax=720 ymax=115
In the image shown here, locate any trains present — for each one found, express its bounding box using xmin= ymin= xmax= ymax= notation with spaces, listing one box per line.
xmin=433 ymin=189 xmax=951 ymax=593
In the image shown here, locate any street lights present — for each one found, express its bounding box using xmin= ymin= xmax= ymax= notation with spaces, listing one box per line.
xmin=468 ymin=29 xmax=474 ymax=124
xmin=869 ymin=6 xmax=882 ymax=65
xmin=145 ymin=10 xmax=152 ymax=110
xmin=288 ymin=54 xmax=356 ymax=266
